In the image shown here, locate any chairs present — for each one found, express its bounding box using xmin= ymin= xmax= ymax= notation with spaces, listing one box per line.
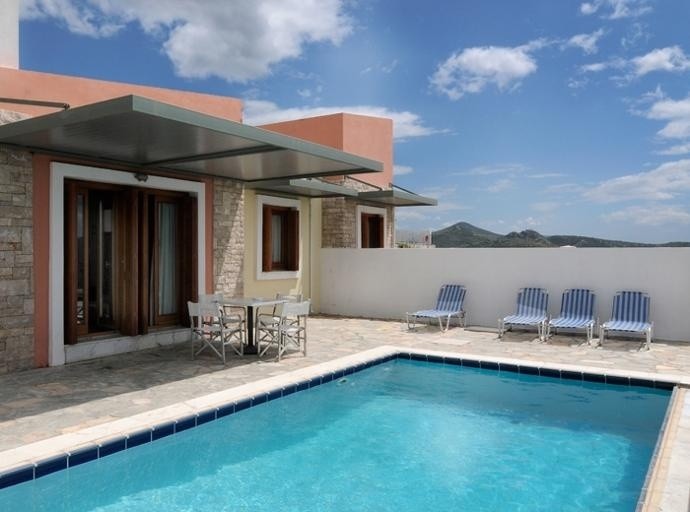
xmin=188 ymin=301 xmax=243 ymax=361
xmin=550 ymin=288 xmax=596 ymax=346
xmin=494 ymin=284 xmax=548 ymax=345
xmin=596 ymin=290 xmax=655 ymax=350
xmin=203 ymin=291 xmax=243 ymax=348
xmin=257 ymin=298 xmax=312 ymax=363
xmin=253 ymin=292 xmax=304 ymax=352
xmin=403 ymin=282 xmax=469 ymax=334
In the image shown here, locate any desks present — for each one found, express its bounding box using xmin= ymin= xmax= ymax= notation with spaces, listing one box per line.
xmin=221 ymin=295 xmax=285 ymax=356
